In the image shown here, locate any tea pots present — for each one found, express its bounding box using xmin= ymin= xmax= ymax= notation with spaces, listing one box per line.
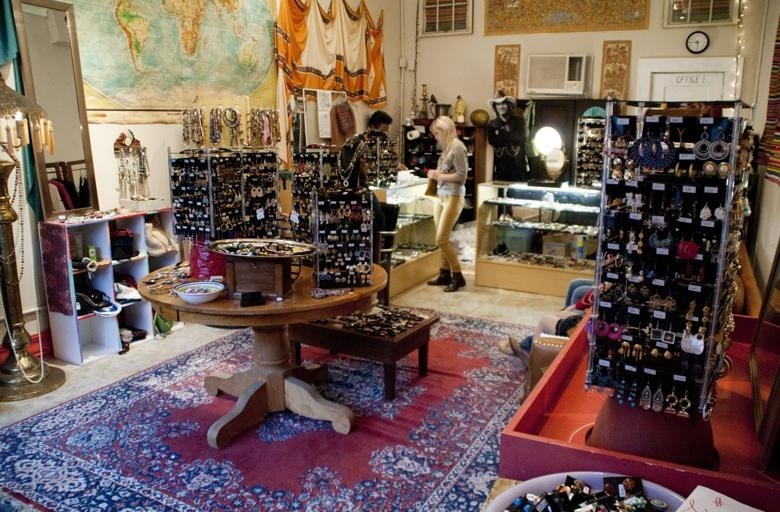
xmin=430 ymin=102 xmax=451 ymax=120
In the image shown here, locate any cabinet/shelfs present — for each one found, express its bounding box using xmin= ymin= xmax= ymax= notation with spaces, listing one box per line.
xmin=397 ymin=120 xmax=491 ymax=223
xmin=471 ymin=175 xmax=602 ymax=300
xmin=278 ymin=184 xmax=446 ymax=300
xmin=35 ymin=196 xmax=192 ymax=369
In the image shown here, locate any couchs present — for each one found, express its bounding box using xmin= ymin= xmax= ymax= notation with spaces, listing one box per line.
xmin=526 ymin=237 xmax=765 ymax=402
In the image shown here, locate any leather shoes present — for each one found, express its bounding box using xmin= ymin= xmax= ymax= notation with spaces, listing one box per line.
xmin=498 ymin=340 xmax=511 ymax=355
xmin=510 ymin=335 xmax=531 ymax=366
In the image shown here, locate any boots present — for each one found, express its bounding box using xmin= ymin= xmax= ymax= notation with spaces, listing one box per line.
xmin=444 ymin=272 xmax=466 ymax=292
xmin=427 ymin=267 xmax=452 ymax=286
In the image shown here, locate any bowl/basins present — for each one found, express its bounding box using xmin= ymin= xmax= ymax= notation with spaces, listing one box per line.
xmin=485 ymin=469 xmax=685 ymax=512
xmin=173 ymin=281 xmax=227 ymax=305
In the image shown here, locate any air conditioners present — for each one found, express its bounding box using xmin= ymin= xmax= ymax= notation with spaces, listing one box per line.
xmin=527 ymin=53 xmax=594 ymax=100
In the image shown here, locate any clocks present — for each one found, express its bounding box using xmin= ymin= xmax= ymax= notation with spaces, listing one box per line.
xmin=684 ymin=30 xmax=711 ymax=55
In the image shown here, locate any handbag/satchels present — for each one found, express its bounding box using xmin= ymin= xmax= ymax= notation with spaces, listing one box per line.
xmin=151 ymin=306 xmax=173 ymax=340
xmin=110 ymin=226 xmax=139 ymax=262
xmin=424 ymin=178 xmax=437 ymax=197
xmin=74 ymin=285 xmax=122 ymax=320
xmin=143 ymin=217 xmax=178 ymax=257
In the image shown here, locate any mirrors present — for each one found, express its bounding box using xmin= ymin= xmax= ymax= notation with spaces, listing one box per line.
xmin=7 ymin=1 xmax=100 ymax=217
xmin=745 ymin=233 xmax=778 ymax=446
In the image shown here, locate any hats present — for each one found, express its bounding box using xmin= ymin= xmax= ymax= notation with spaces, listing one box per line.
xmin=487 ymin=89 xmax=516 ymax=107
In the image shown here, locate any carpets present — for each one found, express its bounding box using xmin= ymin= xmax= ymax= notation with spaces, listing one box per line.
xmin=0 ymin=306 xmax=551 ymax=510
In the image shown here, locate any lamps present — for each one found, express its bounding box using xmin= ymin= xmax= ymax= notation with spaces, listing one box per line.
xmin=0 ymin=68 xmax=69 ymax=413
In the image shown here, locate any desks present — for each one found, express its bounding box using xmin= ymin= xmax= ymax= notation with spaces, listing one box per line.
xmin=471 ymin=301 xmax=780 ymax=512
xmin=284 ymin=300 xmax=442 ymax=407
xmin=132 ymin=250 xmax=392 ymax=453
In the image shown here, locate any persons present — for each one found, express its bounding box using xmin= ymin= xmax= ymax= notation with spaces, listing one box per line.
xmin=489 ymin=89 xmax=528 ymax=181
xmin=497 ymin=278 xmax=595 ymax=366
xmin=428 ymin=116 xmax=469 ymax=292
xmin=366 ymin=110 xmax=406 ymax=171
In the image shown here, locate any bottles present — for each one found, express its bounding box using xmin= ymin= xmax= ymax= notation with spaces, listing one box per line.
xmin=454 ymin=96 xmax=466 ymax=124
